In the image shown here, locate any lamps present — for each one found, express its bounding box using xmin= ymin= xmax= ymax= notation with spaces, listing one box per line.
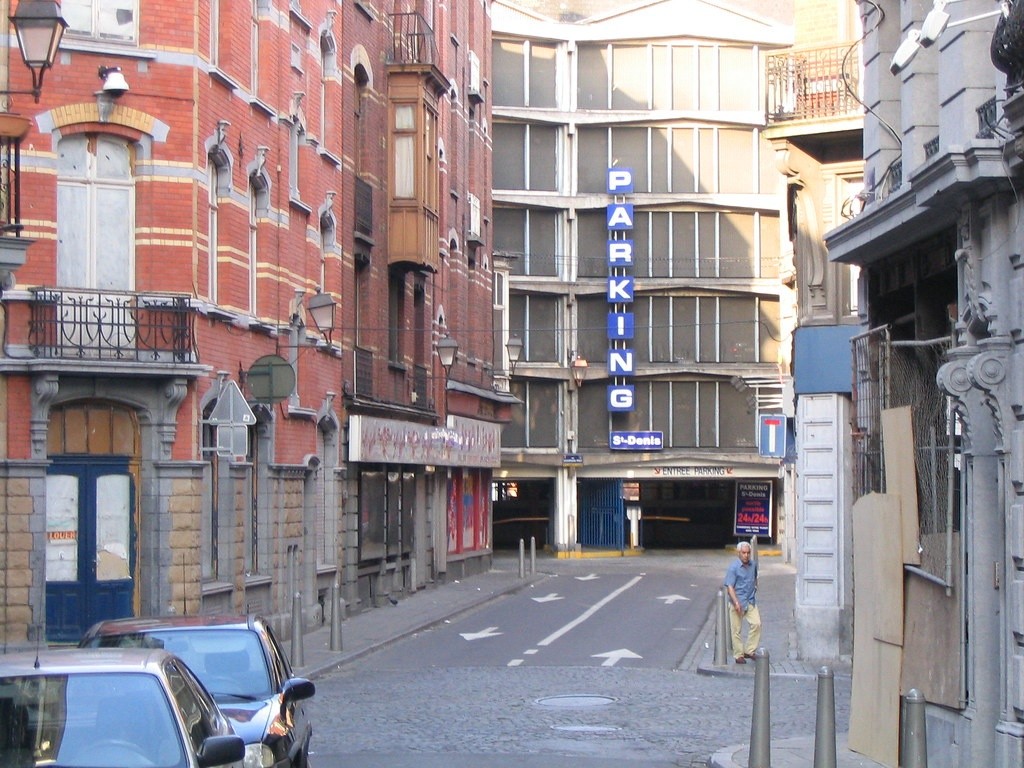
xmin=410 ymin=331 xmax=458 ymax=379
xmin=484 ymin=334 xmax=523 ymax=376
xmin=276 ymin=287 xmax=337 ymax=365
xmin=0 ymin=0 xmax=70 ymax=104
xmin=570 ymin=356 xmax=588 ymax=387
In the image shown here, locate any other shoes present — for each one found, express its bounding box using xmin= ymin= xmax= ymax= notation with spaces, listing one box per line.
xmin=736 ymin=657 xmax=746 ymax=663
xmin=744 ymin=652 xmax=756 ymax=660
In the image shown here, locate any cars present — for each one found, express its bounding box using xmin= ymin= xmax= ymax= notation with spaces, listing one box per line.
xmin=57 ymin=611 xmax=317 ymax=768
xmin=0 ymin=644 xmax=246 ymax=768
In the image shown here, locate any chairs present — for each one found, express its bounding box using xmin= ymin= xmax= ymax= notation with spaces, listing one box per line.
xmin=96 ymin=695 xmax=151 ymax=736
xmin=205 ymin=650 xmax=249 ymax=673
xmin=0 ymin=697 xmax=29 ymax=749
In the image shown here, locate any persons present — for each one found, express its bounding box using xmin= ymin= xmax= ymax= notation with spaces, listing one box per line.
xmin=724 ymin=542 xmax=761 ymax=663
